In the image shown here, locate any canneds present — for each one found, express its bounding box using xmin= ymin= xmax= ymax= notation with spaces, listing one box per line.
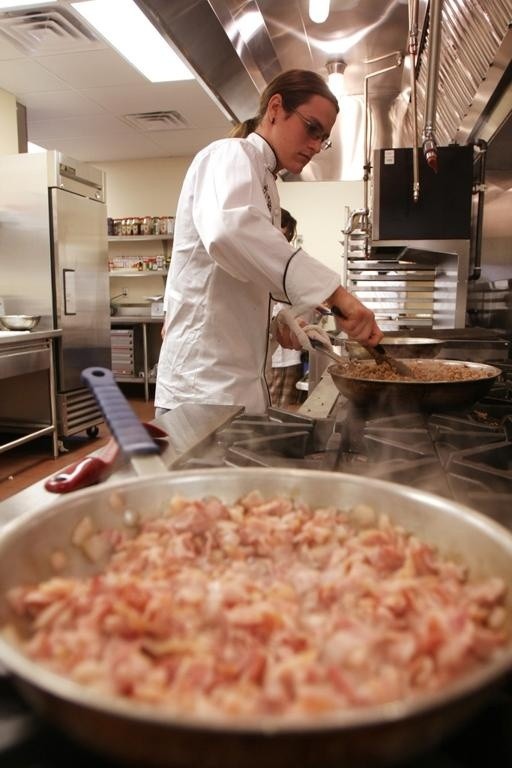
xmin=113 ymin=216 xmax=175 ymax=235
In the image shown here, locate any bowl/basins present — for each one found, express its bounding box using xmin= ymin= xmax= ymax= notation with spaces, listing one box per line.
xmin=1 ymin=315 xmax=41 ymax=333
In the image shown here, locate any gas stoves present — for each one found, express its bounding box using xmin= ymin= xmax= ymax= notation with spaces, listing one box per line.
xmin=0 ymin=402 xmax=511 ymax=766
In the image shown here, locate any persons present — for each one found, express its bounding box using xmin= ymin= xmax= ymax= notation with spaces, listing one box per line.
xmin=265 ymin=208 xmax=331 ymax=406
xmin=139 ymin=70 xmax=384 ymax=424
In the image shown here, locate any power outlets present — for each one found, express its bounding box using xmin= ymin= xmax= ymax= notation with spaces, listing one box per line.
xmin=122 ymin=287 xmax=128 ymax=298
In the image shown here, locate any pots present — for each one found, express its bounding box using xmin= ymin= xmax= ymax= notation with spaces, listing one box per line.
xmin=310 ymin=340 xmax=500 ymax=413
xmin=0 ymin=365 xmax=510 ymax=767
xmin=329 ymin=335 xmax=451 ymax=356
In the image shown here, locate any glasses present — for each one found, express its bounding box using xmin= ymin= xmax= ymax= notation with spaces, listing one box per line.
xmin=281 ymin=99 xmax=333 ymax=152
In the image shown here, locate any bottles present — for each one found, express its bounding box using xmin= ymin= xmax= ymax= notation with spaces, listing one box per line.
xmin=138 ymin=256 xmax=171 ymax=271
xmin=108 ymin=216 xmax=175 ymax=234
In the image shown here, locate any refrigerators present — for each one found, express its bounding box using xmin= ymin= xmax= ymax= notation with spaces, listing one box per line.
xmin=1 ymin=154 xmax=112 ymax=438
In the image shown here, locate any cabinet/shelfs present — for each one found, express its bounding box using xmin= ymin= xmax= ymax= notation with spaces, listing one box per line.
xmin=106 ymin=232 xmax=176 ymax=277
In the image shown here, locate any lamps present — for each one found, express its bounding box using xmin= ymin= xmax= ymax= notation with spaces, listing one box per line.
xmin=324 ymin=58 xmax=352 ymax=99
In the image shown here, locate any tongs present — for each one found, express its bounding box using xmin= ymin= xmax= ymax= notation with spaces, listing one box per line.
xmin=332 ymin=310 xmax=412 ymax=376
